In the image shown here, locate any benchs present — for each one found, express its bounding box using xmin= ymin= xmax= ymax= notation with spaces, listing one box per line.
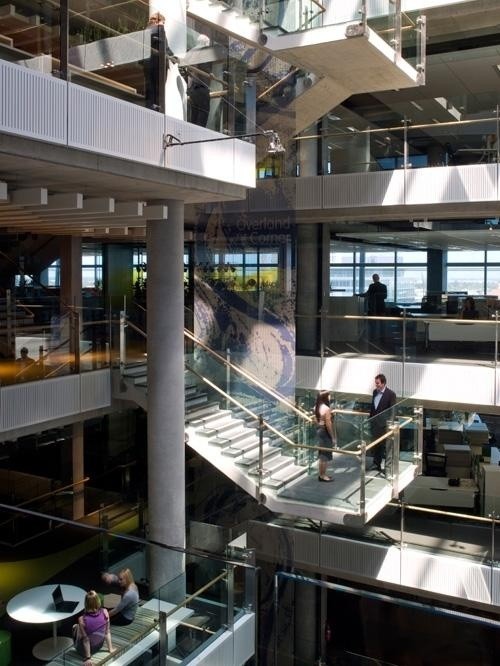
xmin=46 ymin=605 xmax=162 ymax=666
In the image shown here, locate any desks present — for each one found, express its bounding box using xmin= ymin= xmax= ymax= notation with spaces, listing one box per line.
xmin=406 ymin=418 xmax=500 ymax=530
xmin=396 ymin=300 xmax=500 ymax=359
xmin=5 ymin=582 xmax=89 ymax=661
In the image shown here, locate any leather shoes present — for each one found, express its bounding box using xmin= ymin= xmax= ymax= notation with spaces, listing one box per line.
xmin=368 ymin=464 xmax=381 ymax=471
xmin=319 ymin=475 xmax=334 ymax=481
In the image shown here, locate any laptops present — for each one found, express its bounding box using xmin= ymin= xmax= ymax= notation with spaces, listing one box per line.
xmin=52 ymin=585 xmax=79 ymax=613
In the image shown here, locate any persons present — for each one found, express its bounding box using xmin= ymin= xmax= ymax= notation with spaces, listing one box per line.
xmin=105 ymin=567 xmax=139 ymax=627
xmin=73 ymin=591 xmax=116 ymax=666
xmin=314 ymin=390 xmax=336 ymax=482
xmin=461 ymin=296 xmax=479 ymax=319
xmin=369 ymin=374 xmax=396 ymax=473
xmin=366 ymin=274 xmax=387 ymax=341
xmin=179 ymin=34 xmax=215 ymax=127
xmin=142 ymin=14 xmax=179 ymax=111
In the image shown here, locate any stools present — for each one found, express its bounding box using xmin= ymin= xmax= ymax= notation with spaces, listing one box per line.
xmin=0 ymin=629 xmax=14 ymax=665
